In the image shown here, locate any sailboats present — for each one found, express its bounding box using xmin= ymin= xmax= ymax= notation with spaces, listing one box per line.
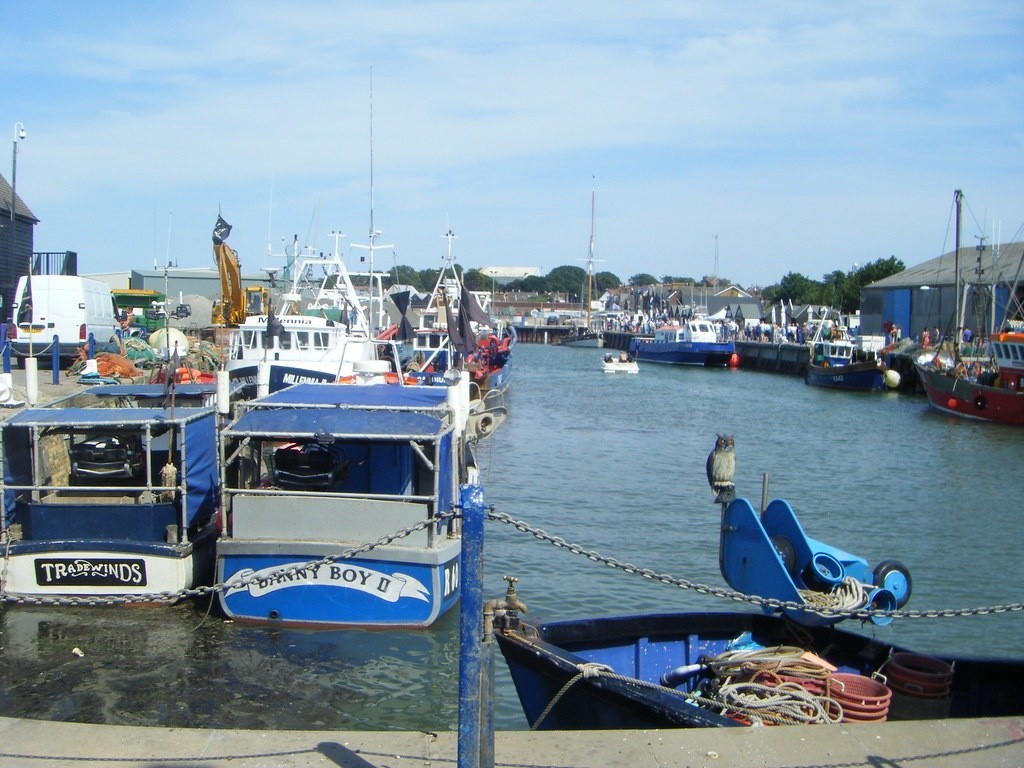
xmin=559 ymin=173 xmax=606 ymax=349
xmin=911 ymin=188 xmax=1024 ymax=427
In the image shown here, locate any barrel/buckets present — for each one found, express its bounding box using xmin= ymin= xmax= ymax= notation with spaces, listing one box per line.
xmin=886 ymin=647 xmax=955 ymax=700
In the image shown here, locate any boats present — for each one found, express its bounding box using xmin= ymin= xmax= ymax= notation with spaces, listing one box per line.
xmin=627 ymin=316 xmax=736 ymax=368
xmin=491 ymin=470 xmax=1024 ymax=730
xmin=803 ymin=309 xmax=907 ymax=390
xmin=601 ymin=354 xmax=640 ymax=374
xmin=1 ymin=65 xmax=522 ymax=631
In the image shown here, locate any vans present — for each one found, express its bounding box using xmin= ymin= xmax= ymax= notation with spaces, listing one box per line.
xmin=7 ymin=274 xmax=128 ymax=369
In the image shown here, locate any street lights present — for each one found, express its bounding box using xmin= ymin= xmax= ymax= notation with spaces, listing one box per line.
xmin=9 ymin=120 xmax=27 ymax=321
xmin=921 ymin=285 xmax=942 ymax=335
xmin=490 ymin=271 xmax=498 ymax=317
